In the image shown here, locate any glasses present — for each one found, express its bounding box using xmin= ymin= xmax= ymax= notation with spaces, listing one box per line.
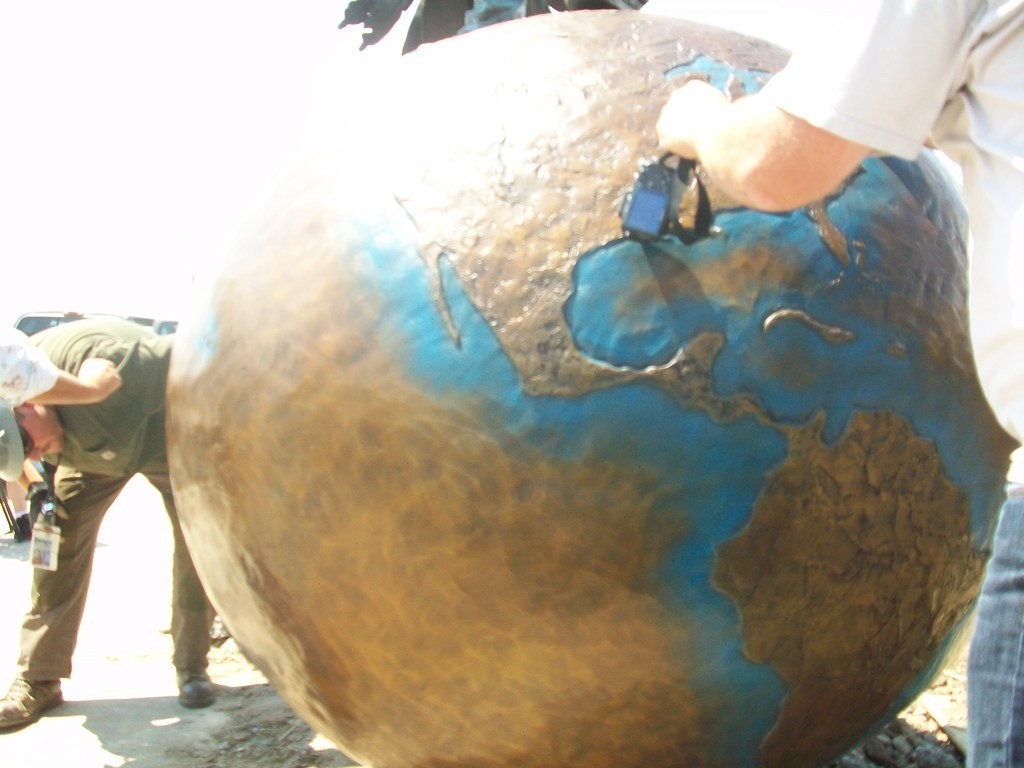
xmin=17 ymin=425 xmax=34 ymax=460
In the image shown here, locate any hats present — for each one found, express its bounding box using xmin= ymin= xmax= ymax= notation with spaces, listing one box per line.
xmin=0 ymin=395 xmax=25 ymax=483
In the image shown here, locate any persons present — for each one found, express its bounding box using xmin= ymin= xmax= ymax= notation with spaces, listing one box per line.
xmin=656 ymin=0 xmax=1024 ymax=768
xmin=0 ymin=309 xmax=223 ymax=737
xmin=337 ymin=0 xmax=650 ymax=57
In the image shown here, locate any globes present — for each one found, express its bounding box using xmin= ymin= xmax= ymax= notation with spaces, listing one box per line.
xmin=163 ymin=9 xmax=1022 ymax=768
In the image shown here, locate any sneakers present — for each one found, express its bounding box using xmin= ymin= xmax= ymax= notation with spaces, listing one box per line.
xmin=0 ymin=677 xmax=63 ymax=727
xmin=177 ymin=667 xmax=214 ymax=707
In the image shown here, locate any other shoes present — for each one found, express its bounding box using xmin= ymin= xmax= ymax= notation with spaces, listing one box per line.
xmin=13 ymin=513 xmax=31 ymax=543
